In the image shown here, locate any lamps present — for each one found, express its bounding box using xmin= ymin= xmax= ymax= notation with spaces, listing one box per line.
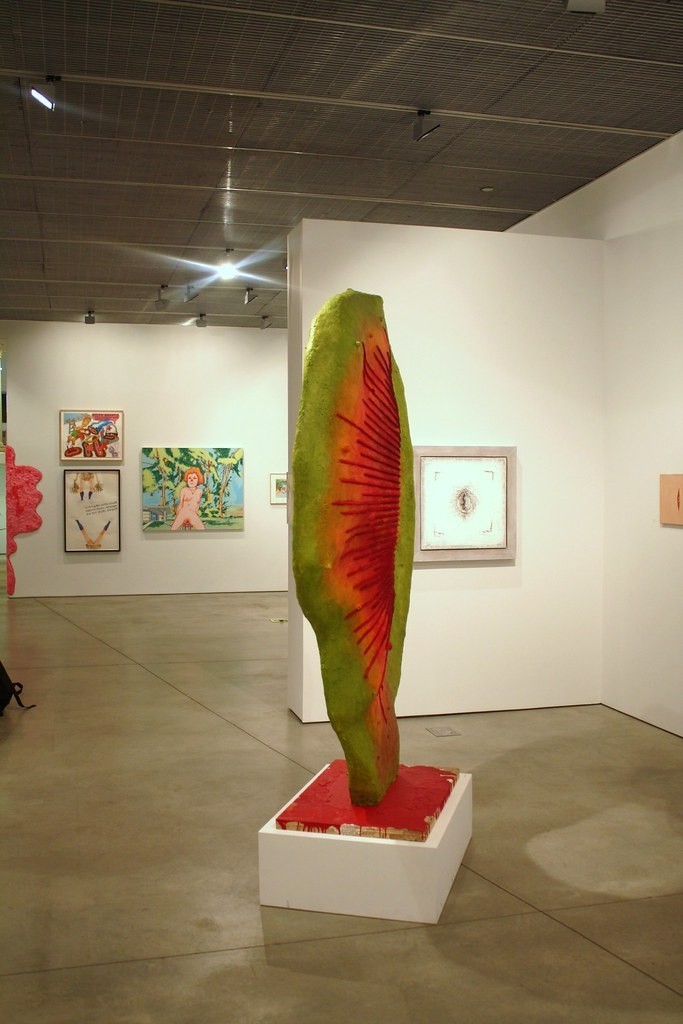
xmin=412 ymin=110 xmax=440 ymax=141
xmin=84 ymin=250 xmax=285 ymax=330
xmin=29 ymin=74 xmax=62 ymax=112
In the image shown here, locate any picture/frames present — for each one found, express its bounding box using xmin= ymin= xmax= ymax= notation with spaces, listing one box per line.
xmin=64 ymin=469 xmax=121 ymax=552
xmin=270 ymin=473 xmax=287 ymax=505
xmin=60 ymin=409 xmax=124 ymax=461
xmin=412 ymin=445 xmax=517 ymax=563
